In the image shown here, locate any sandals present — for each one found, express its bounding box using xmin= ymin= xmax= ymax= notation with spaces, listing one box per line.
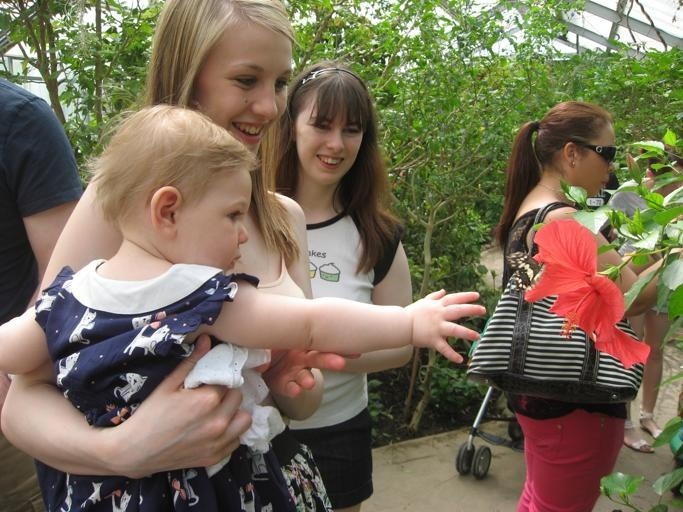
xmin=622 ymin=419 xmax=656 ymax=455
xmin=638 ymin=409 xmax=663 ymax=440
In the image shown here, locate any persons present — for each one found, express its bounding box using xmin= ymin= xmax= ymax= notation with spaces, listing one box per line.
xmin=0 ymin=0 xmax=362 ymax=512
xmin=595 ymin=144 xmax=683 ymax=452
xmin=0 ymin=106 xmax=486 ymax=512
xmin=248 ymin=61 xmax=414 ymax=512
xmin=495 ymin=101 xmax=683 ymax=512
xmin=584 ymin=171 xmax=622 ymax=242
xmin=0 ymin=65 xmax=83 ymax=512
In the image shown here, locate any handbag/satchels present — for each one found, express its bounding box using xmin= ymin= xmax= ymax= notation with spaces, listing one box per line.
xmin=464 ymin=254 xmax=646 ymax=406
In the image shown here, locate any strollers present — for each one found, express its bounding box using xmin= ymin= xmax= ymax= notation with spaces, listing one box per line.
xmin=453 ymin=382 xmax=523 ymax=483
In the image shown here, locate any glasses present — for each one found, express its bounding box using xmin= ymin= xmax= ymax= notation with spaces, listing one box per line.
xmin=582 ymin=142 xmax=618 ymax=164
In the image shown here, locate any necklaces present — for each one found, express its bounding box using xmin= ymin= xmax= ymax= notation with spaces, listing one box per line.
xmin=536 ymin=182 xmax=562 ymax=192
